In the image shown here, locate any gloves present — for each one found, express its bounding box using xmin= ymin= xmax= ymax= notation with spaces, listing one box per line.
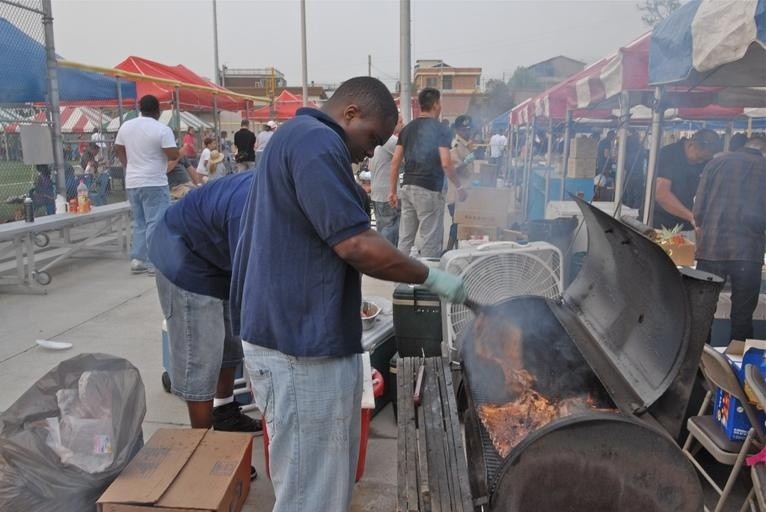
xmin=422 ymin=266 xmax=468 ymax=305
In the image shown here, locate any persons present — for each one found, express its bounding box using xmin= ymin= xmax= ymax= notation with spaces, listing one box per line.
xmin=233 ymin=119 xmax=257 ymax=173
xmin=53 ymin=150 xmax=77 ymax=199
xmin=114 ymin=95 xmax=179 ymax=277
xmin=166 ymin=150 xmax=202 ymax=198
xmin=208 ymin=150 xmax=227 ymax=182
xmin=197 ymin=138 xmax=216 ymax=180
xmin=371 ymin=111 xmax=406 ymax=247
xmin=255 ymin=122 xmax=278 ymax=165
xmin=446 ymin=114 xmax=485 ymax=249
xmin=693 ymin=136 xmax=766 ymax=344
xmin=147 ymin=168 xmax=264 ymax=481
xmin=388 ymin=88 xmax=467 ymax=259
xmin=488 ymin=127 xmax=510 ymax=165
xmin=232 ymin=76 xmax=467 ymax=512
xmin=82 ymin=144 xmax=112 ymax=192
xmin=60 ymin=128 xmax=106 ymax=158
xmin=183 ymin=125 xmax=201 ymax=167
xmin=597 ymin=129 xmax=643 ymax=209
xmin=24 ymin=163 xmax=55 ymax=210
xmin=220 ymin=130 xmax=234 ymax=163
xmin=652 ymin=128 xmax=719 ymax=234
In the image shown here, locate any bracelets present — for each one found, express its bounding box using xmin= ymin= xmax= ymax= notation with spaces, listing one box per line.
xmin=455 ymin=185 xmax=464 ymax=191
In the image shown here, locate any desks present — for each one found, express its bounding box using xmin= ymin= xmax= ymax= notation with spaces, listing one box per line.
xmin=0 ymin=201 xmax=135 ymax=297
xmin=545 ymin=200 xmax=639 ymax=221
xmin=527 ymin=170 xmax=594 ymax=221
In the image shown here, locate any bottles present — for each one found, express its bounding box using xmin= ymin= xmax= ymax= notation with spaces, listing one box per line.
xmin=77 ymin=180 xmax=91 ymax=212
xmin=23 ymin=197 xmax=34 ymax=222
xmin=69 ymin=197 xmax=77 ymax=212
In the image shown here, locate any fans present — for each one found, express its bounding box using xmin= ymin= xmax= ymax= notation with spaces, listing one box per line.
xmin=438 ymin=240 xmax=565 ymax=359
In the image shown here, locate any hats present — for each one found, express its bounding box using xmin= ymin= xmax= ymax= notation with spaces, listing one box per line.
xmin=455 ymin=115 xmax=472 ymax=129
xmin=264 ymin=121 xmax=276 ymax=129
xmin=209 ymin=150 xmax=224 ymax=163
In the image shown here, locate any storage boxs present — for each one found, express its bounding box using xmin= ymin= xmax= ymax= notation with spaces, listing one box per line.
xmin=160 ymin=319 xmax=250 ymax=404
xmin=354 ymin=292 xmax=392 ymax=421
xmin=569 ymin=138 xmax=597 ymax=159
xmin=95 ymin=426 xmax=254 ymax=512
xmin=390 ymin=352 xmax=466 ymax=428
xmin=567 ymin=158 xmax=596 ymax=178
xmin=393 ymin=255 xmax=442 ymax=359
xmin=453 ymin=188 xmax=509 ymax=228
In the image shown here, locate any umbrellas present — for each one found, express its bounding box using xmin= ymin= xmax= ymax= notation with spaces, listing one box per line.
xmin=4 ymin=107 xmax=112 ymax=132
xmin=34 ymin=57 xmax=254 ymax=111
xmin=96 ymin=109 xmax=217 ymax=131
xmin=0 ymin=17 xmax=136 ymax=103
xmin=243 ymin=87 xmax=317 ymax=119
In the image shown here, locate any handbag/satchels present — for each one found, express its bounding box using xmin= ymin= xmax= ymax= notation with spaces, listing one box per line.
xmin=235 ymin=151 xmax=249 ymax=162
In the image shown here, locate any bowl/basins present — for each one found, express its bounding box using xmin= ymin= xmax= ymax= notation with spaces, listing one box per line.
xmin=362 ymin=298 xmax=381 ymax=331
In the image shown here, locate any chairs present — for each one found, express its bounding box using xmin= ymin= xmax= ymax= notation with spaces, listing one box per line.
xmin=679 ymin=343 xmax=766 ymax=512
xmin=87 ymin=173 xmax=110 ymax=206
xmin=741 ymin=346 xmax=766 ymax=512
xmin=75 ymin=173 xmax=93 ymax=194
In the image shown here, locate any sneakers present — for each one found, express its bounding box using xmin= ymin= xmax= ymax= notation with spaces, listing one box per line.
xmin=250 ymin=464 xmax=257 ymax=479
xmin=131 ymin=259 xmax=147 ymax=273
xmin=146 ymin=263 xmax=156 ymax=275
xmin=211 ymin=396 xmax=263 ymax=437
